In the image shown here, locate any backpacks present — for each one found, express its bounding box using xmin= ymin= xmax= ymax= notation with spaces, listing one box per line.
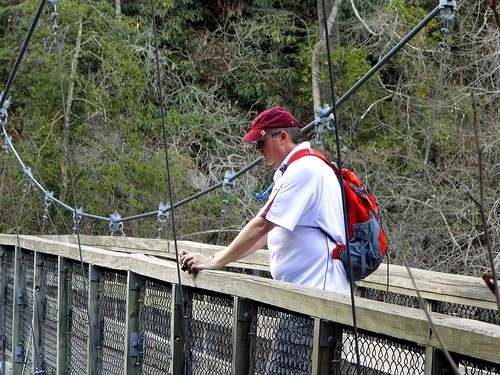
xmin=284 ymin=153 xmax=389 ymax=283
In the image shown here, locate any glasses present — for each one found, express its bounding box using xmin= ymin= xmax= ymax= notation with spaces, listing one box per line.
xmin=251 ymin=130 xmax=283 ymax=150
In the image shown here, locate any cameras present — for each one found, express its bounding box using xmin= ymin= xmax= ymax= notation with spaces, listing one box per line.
xmin=180 ymin=257 xmax=202 ymax=272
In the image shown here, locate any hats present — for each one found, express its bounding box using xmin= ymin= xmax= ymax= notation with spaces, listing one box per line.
xmin=240 ymin=106 xmax=301 ymax=141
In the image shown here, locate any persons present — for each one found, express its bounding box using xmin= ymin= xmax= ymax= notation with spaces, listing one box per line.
xmin=178 ymin=105 xmax=353 ymax=374
